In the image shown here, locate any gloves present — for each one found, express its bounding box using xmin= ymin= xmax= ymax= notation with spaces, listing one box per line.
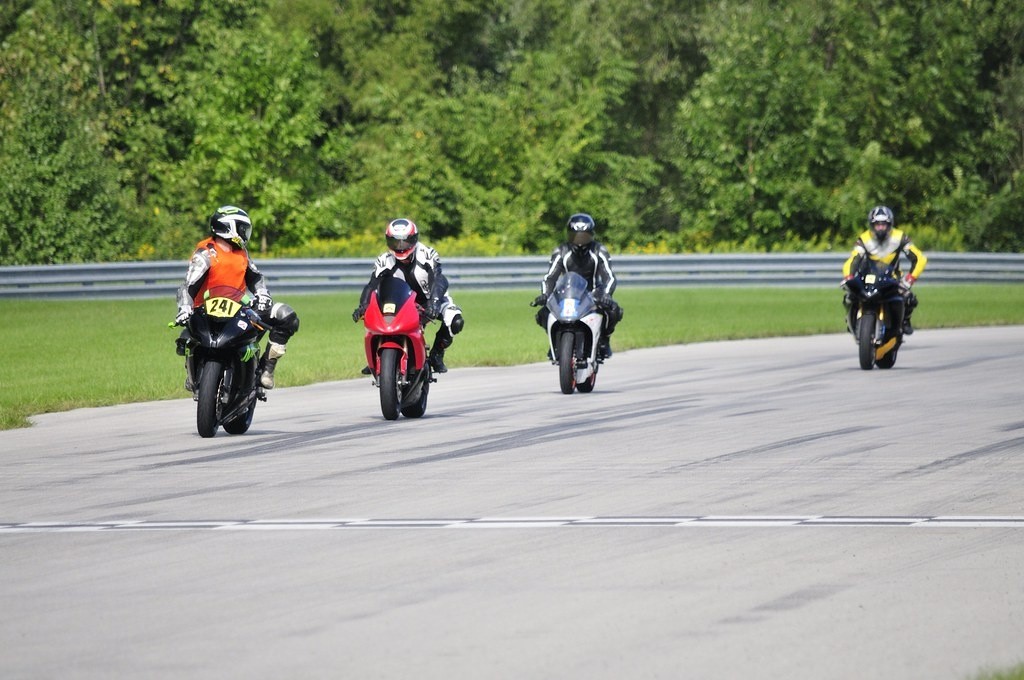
xmin=422 ymin=297 xmax=441 ymax=319
xmin=353 ymin=305 xmax=366 ymax=322
xmin=899 ymin=274 xmax=916 ymax=290
xmin=175 ymin=305 xmax=192 ymax=327
xmin=535 ymin=294 xmax=547 ymax=305
xmin=255 ymin=289 xmax=273 ymax=311
xmin=600 ymin=294 xmax=612 ymax=308
xmin=840 ymin=273 xmax=854 ymax=291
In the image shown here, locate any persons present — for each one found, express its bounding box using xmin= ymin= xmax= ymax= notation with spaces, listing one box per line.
xmin=842 ymin=206 xmax=926 ymax=334
xmin=352 ymin=218 xmax=463 ymax=372
xmin=175 ymin=206 xmax=299 ymax=391
xmin=535 ymin=214 xmax=619 ymax=361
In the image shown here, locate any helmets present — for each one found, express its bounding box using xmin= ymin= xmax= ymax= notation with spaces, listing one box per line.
xmin=210 ymin=206 xmax=251 ymax=251
xmin=567 ymin=214 xmax=595 ymax=257
xmin=385 ymin=219 xmax=418 ymax=261
xmin=869 ymin=206 xmax=894 ymax=243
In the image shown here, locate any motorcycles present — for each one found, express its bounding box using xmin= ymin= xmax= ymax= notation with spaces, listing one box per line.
xmin=167 ymin=286 xmax=269 ymax=438
xmin=530 ymin=273 xmax=610 ymax=395
xmin=839 ymin=254 xmax=909 ymax=370
xmin=355 ymin=277 xmax=442 ymax=420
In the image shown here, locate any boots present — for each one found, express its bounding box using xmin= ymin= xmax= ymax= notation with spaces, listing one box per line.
xmin=255 ymin=339 xmax=286 ymax=388
xmin=428 ymin=330 xmax=452 ymax=373
xmin=902 ymin=307 xmax=915 ymax=334
xmin=599 ymin=327 xmax=614 ymax=356
xmin=185 ymin=376 xmax=193 ymax=391
xmin=362 ymin=366 xmax=371 ymax=374
xmin=847 ymin=305 xmax=860 ymax=332
xmin=547 ymin=350 xmax=553 ymax=358
